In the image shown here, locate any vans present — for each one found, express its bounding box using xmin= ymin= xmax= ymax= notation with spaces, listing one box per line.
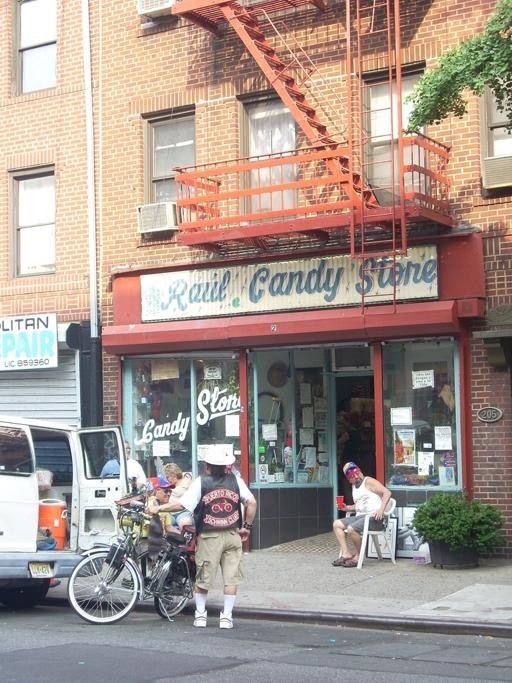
xmin=0 ymin=421 xmax=146 ymax=607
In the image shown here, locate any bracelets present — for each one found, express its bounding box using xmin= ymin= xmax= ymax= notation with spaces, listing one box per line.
xmin=242 ymin=522 xmax=253 ymax=530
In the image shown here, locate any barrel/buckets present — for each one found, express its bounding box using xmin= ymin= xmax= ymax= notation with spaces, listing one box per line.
xmin=39 ymin=500 xmax=68 ymax=550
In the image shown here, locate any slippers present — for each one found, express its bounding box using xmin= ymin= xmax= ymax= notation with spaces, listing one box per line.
xmin=332 ymin=555 xmax=363 ymax=567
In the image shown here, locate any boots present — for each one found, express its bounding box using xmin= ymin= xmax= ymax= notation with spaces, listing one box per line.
xmin=219 ymin=610 xmax=233 ymax=629
xmin=193 ymin=609 xmax=207 ymax=627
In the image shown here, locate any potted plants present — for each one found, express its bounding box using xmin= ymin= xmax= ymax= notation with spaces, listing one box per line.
xmin=407 ymin=491 xmax=507 ymax=568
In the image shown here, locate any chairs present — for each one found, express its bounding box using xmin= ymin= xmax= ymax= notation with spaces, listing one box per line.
xmin=339 ymin=497 xmax=397 ymax=569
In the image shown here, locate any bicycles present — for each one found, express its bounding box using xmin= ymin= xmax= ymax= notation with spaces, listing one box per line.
xmin=67 ymin=498 xmax=197 ymax=625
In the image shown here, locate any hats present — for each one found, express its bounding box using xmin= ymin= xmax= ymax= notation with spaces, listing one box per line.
xmin=152 ymin=477 xmax=175 ymax=489
xmin=204 ymin=444 xmax=235 ymax=466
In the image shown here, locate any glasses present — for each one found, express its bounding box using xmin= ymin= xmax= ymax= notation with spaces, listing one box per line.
xmin=159 ymin=489 xmax=173 ymax=494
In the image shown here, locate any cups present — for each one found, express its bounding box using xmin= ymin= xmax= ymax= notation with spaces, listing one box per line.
xmin=336 ymin=496 xmax=344 ymax=510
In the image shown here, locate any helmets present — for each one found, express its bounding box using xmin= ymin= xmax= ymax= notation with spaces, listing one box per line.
xmin=343 ymin=462 xmax=360 ymax=480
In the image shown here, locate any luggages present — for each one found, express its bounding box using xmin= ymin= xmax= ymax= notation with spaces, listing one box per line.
xmin=251 ymin=397 xmax=284 ymax=471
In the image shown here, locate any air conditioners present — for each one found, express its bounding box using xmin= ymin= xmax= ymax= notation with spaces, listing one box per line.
xmin=137 ymin=202 xmax=178 ymax=235
xmin=137 ymin=0 xmax=175 ymax=18
xmin=482 ymin=156 xmax=512 ymax=190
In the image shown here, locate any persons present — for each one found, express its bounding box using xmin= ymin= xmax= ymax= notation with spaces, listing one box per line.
xmin=100 ymin=440 xmax=192 ymax=556
xmin=144 ymin=463 xmax=257 ymax=629
xmin=337 ymin=413 xmax=361 ymax=504
xmin=331 ymin=462 xmax=392 ymax=567
